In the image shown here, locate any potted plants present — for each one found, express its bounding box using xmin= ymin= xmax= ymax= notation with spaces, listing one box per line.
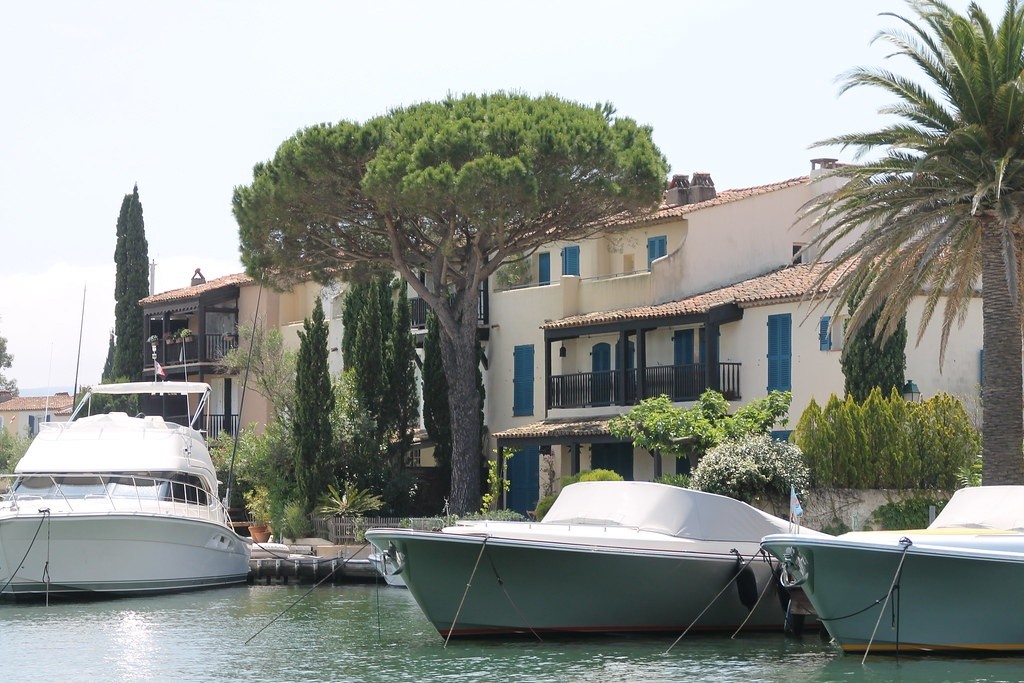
xmin=164 ymin=334 xmax=174 ymax=344
xmin=243 ymin=486 xmax=272 ymax=541
xmin=174 ymin=328 xmax=185 ymax=344
xmin=147 ymin=335 xmax=159 ymax=346
xmin=221 ymin=332 xmax=234 ymax=341
xmin=180 ymin=329 xmax=193 ymax=343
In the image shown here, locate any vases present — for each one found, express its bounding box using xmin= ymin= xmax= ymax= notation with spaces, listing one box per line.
xmin=254 ymin=531 xmax=271 ymax=542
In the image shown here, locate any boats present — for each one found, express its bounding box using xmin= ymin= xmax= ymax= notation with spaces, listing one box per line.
xmin=753 ymin=483 xmax=1024 ymax=659
xmin=367 ymin=551 xmax=409 ymax=588
xmin=245 ymin=541 xmax=290 ymax=560
xmin=0 ymin=381 xmax=251 ymax=595
xmin=364 ymin=479 xmax=841 ymax=644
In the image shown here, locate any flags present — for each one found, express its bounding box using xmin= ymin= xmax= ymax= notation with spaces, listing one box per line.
xmin=790 ymin=484 xmax=804 ymax=517
xmin=154 ymin=360 xmax=165 ymax=377
